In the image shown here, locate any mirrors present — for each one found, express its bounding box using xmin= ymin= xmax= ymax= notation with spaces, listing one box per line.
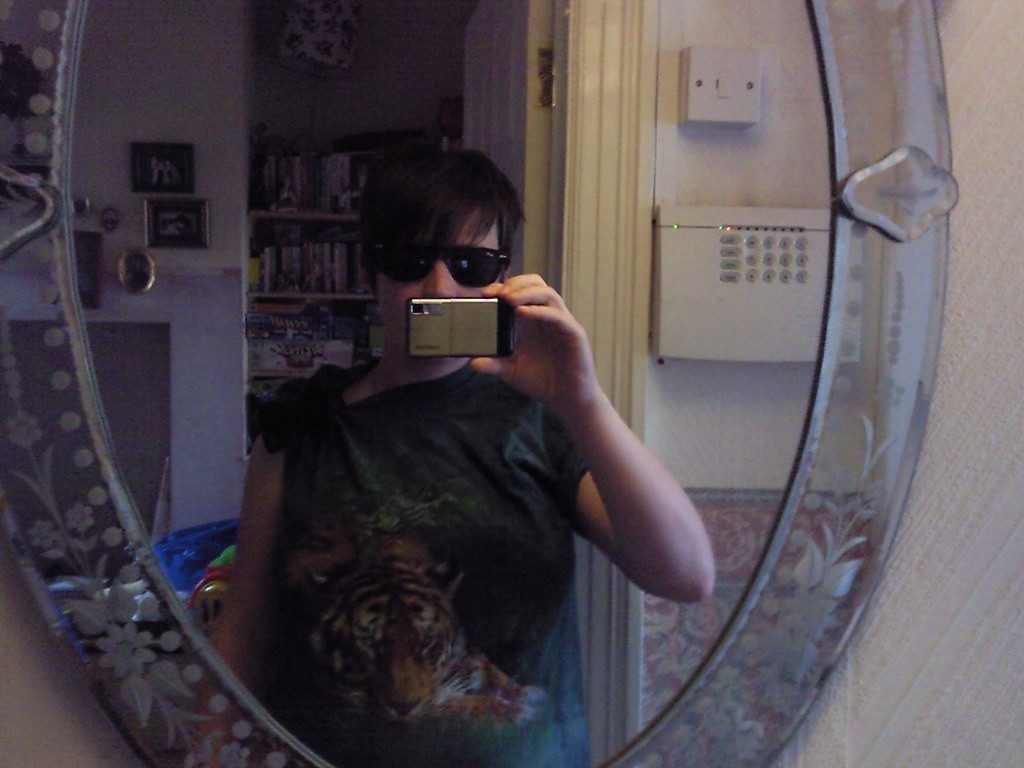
xmin=1 ymin=2 xmax=959 ymax=768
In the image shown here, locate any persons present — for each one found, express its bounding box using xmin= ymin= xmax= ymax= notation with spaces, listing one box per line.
xmin=148 ymin=159 xmax=183 ymax=186
xmin=193 ymin=148 xmax=715 ymax=768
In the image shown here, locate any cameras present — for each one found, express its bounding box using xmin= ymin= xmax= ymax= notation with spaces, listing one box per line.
xmin=405 ymin=296 xmax=514 ymax=358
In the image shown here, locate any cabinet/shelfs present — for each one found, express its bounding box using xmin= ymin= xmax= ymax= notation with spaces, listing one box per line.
xmin=247 ymin=206 xmax=381 ymax=380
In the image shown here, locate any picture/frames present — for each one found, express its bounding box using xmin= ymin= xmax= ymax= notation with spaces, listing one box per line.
xmin=101 ymin=207 xmax=118 ymax=232
xmin=143 ymin=198 xmax=210 ymax=252
xmin=117 ymin=247 xmax=155 ymax=293
xmin=74 ymin=231 xmax=102 ymax=309
xmin=73 ymin=196 xmax=92 ymax=215
xmin=123 ymin=141 xmax=195 ymax=198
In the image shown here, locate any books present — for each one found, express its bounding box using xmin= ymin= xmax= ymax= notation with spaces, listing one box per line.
xmin=260 ymin=240 xmax=371 ymax=294
xmin=263 ymin=153 xmax=355 ymax=212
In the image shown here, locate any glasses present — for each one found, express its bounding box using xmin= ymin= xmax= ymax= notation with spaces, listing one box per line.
xmin=368 ymin=240 xmax=514 ymax=286
